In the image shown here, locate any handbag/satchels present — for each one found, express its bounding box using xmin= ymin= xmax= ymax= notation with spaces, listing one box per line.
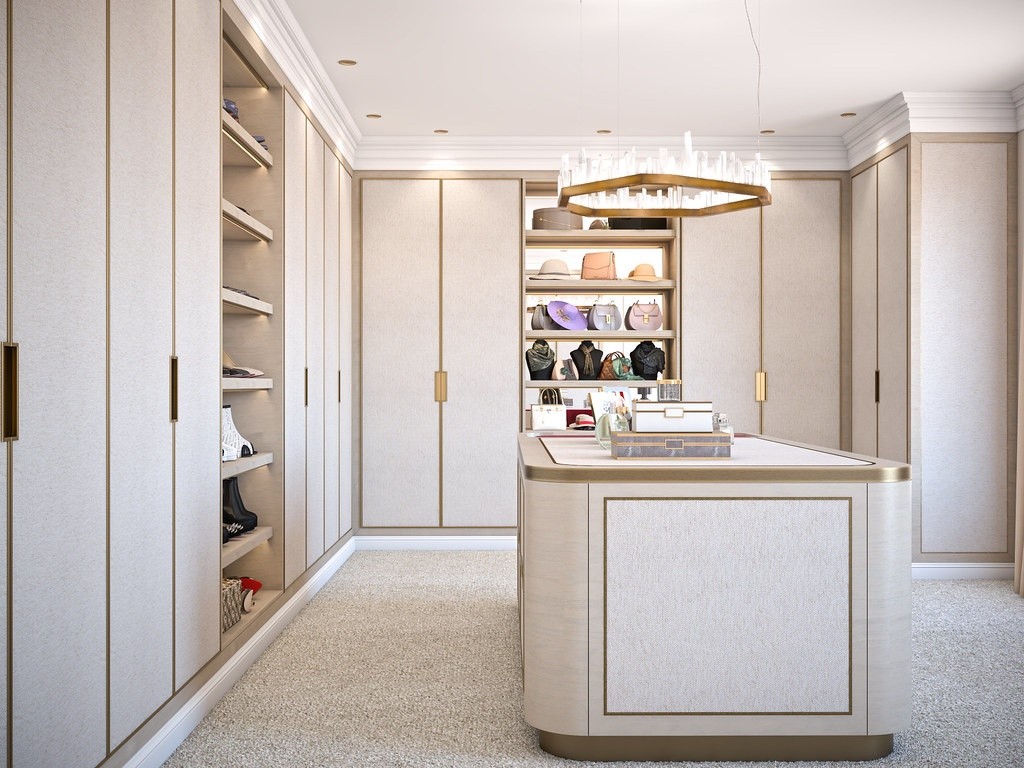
xmin=589 ymin=391 xmax=632 ymax=430
xmin=589 ymin=301 xmax=621 ymax=330
xmin=532 ymin=388 xmax=566 ymax=431
xmin=533 ymin=305 xmax=566 ymax=330
xmin=552 ymin=359 xmax=580 ymax=380
xmin=533 ymin=208 xmax=582 ymax=229
xmin=629 ymin=299 xmax=662 ymax=330
xmin=581 ymin=252 xmax=617 ymax=280
xmin=598 ymin=352 xmax=630 ymax=380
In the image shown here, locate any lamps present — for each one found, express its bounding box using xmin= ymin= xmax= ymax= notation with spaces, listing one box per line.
xmin=557 ymin=0 xmax=773 ymax=218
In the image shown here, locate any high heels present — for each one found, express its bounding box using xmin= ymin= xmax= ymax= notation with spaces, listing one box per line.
xmin=223 ymin=352 xmax=265 ymax=378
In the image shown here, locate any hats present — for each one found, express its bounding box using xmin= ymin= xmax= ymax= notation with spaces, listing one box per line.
xmin=569 ymin=414 xmax=596 ymax=428
xmin=627 ymin=264 xmax=664 ymax=282
xmin=547 ymin=301 xmax=588 ymax=331
xmin=529 ymin=260 xmax=575 ymax=280
xmin=590 ymin=220 xmax=607 ymax=229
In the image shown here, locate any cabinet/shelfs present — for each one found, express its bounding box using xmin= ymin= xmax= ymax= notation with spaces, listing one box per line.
xmin=680 ymin=177 xmax=842 ymax=449
xmin=106 ymin=0 xmax=222 ymax=768
xmin=220 ymin=0 xmax=285 ymax=662
xmin=324 ymin=128 xmax=354 ymax=564
xmin=521 ymin=178 xmax=681 ymax=431
xmin=359 ymin=177 xmax=519 ymax=537
xmin=0 ymin=0 xmax=109 ymax=768
xmin=847 ymin=133 xmax=1017 ymax=564
xmin=286 ymin=76 xmax=325 ymax=601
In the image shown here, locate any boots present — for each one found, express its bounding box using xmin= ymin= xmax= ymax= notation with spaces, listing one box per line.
xmin=223 ymin=477 xmax=258 ymax=531
xmin=221 ymin=405 xmax=257 ymax=462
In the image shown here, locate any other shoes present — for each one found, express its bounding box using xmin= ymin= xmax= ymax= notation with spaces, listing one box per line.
xmin=261 ymin=145 xmax=268 ymax=150
xmin=223 ymin=509 xmax=246 ymax=544
xmin=252 ymin=136 xmax=265 ymax=143
xmin=223 ymin=101 xmax=239 ymax=122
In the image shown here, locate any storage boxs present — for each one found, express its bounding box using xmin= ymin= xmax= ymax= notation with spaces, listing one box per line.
xmin=631 ymin=399 xmax=713 ymax=434
xmin=610 ymin=431 xmax=732 ymax=461
